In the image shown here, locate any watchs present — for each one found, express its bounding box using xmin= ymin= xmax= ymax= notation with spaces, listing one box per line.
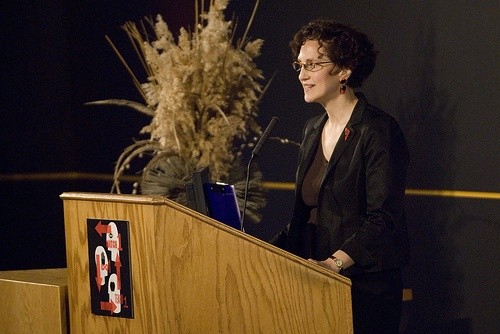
xmin=328 ymin=255 xmax=344 ymax=273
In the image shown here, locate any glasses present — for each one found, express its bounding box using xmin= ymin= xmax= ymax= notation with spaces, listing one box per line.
xmin=292 ymin=60 xmax=335 ymax=72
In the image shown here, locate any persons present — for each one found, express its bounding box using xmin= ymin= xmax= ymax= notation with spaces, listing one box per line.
xmin=262 ymin=21 xmax=403 ymax=333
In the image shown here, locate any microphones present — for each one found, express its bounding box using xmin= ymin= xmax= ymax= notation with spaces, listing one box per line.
xmin=248 ymin=117 xmax=279 ymax=166
